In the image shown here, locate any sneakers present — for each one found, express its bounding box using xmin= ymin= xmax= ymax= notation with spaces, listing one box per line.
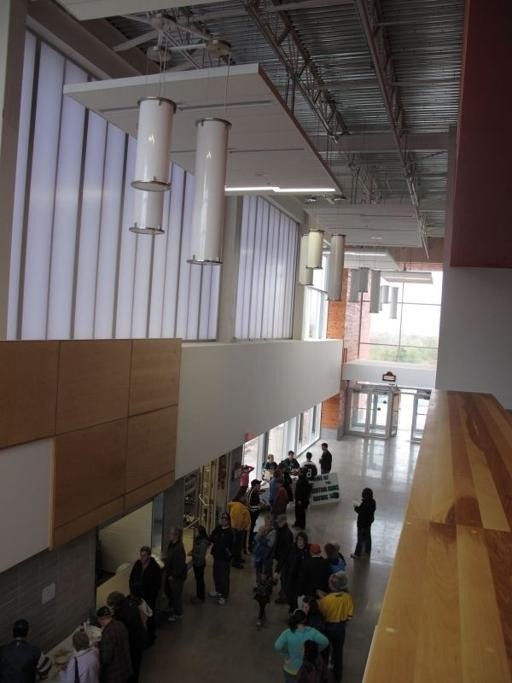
xmin=209 ymin=592 xmax=223 ymax=597
xmin=218 ymin=596 xmax=226 ymax=605
xmin=168 ymin=614 xmax=183 ymax=621
xmin=256 ymin=622 xmax=261 ymax=630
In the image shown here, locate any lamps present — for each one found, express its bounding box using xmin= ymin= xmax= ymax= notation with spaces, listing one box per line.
xmin=325 ymin=228 xmax=349 ymax=304
xmin=129 ymin=11 xmax=183 ymax=195
xmin=347 ymin=265 xmax=401 ymax=319
xmin=303 ymin=191 xmax=327 ymax=271
xmin=295 ymin=205 xmax=318 ymax=287
xmin=126 ymin=188 xmax=169 ymax=238
xmin=181 ymin=31 xmax=242 ymax=271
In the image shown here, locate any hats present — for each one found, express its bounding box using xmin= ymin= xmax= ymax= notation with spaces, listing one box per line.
xmin=308 ymin=543 xmax=321 ymax=554
xmin=97 ymin=606 xmax=110 ymax=616
xmin=251 ymin=479 xmax=261 ymax=486
xmin=329 ymin=571 xmax=348 ymax=591
xmin=275 ymin=514 xmax=287 ymax=523
xmin=14 ymin=619 xmax=28 ymax=629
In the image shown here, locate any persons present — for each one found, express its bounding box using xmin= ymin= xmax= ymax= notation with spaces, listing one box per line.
xmin=352 ymin=487 xmax=377 ymax=558
xmin=106 ymin=451 xmax=355 ymax=683
xmin=95 ymin=604 xmax=135 ymax=681
xmin=58 ymin=629 xmax=99 ymax=681
xmin=1 ymin=617 xmax=53 ymax=682
xmin=318 ymin=442 xmax=333 ymax=474
